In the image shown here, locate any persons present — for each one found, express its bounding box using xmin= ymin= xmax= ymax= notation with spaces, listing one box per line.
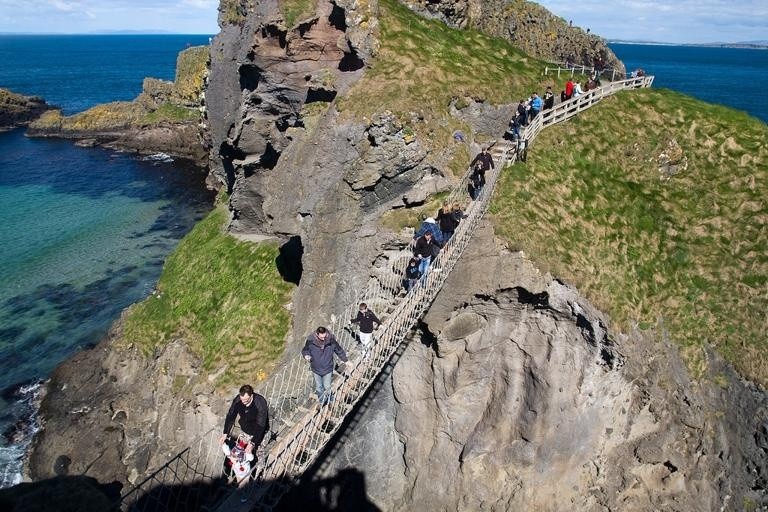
xmin=509 ymin=74 xmax=601 ymax=142
xmin=221 ymin=432 xmax=255 ymax=503
xmin=303 ymin=325 xmax=349 ymax=411
xmin=628 ymin=69 xmax=644 ymax=87
xmin=220 ymin=384 xmax=269 ymax=487
xmin=406 ymin=199 xmax=463 ymax=294
xmin=349 ymin=302 xmax=382 ymax=359
xmin=469 ymin=148 xmax=494 ymax=199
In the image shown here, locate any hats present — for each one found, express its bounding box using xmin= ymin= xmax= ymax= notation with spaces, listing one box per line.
xmin=228 ymin=446 xmax=245 ymax=471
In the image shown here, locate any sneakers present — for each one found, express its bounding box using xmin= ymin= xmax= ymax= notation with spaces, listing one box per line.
xmin=241 ymin=495 xmax=247 ymax=502
